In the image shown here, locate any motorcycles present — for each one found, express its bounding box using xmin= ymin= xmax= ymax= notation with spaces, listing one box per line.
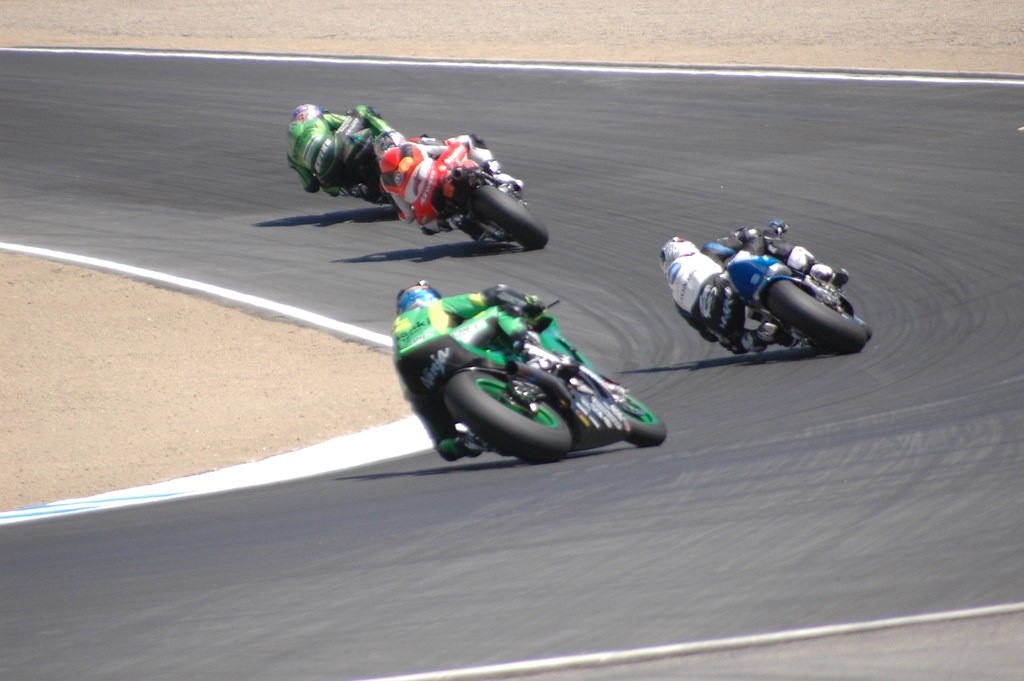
xmin=398 ymin=134 xmax=548 ymax=251
xmin=416 ymin=283 xmax=668 ymax=463
xmin=708 ymin=217 xmax=872 ymax=357
xmin=337 ymin=124 xmax=405 ymax=206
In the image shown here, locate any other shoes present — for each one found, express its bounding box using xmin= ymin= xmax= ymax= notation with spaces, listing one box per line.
xmin=447 ymin=214 xmax=463 ymax=228
xmin=495 ymin=172 xmax=523 ymax=188
xmin=352 ymin=182 xmax=369 ymax=197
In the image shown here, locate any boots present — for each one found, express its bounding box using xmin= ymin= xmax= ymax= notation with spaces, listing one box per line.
xmin=740 ymin=332 xmax=766 ymax=353
xmin=436 ymin=437 xmax=481 ymax=461
xmin=788 ymin=245 xmax=849 ymax=287
xmin=523 ymin=330 xmax=562 ymax=364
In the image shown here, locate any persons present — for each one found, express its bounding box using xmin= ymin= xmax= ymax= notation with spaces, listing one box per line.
xmin=389 ymin=282 xmax=570 ymax=464
xmin=286 ymin=104 xmax=522 ymax=238
xmin=659 ymin=220 xmax=848 ymax=362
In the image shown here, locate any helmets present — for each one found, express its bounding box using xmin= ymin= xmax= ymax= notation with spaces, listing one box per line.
xmin=292 ymin=104 xmax=322 ymax=121
xmin=660 ymin=236 xmax=700 ymax=272
xmin=373 ymin=129 xmax=405 ymax=159
xmin=398 ymin=284 xmax=440 ymax=315
